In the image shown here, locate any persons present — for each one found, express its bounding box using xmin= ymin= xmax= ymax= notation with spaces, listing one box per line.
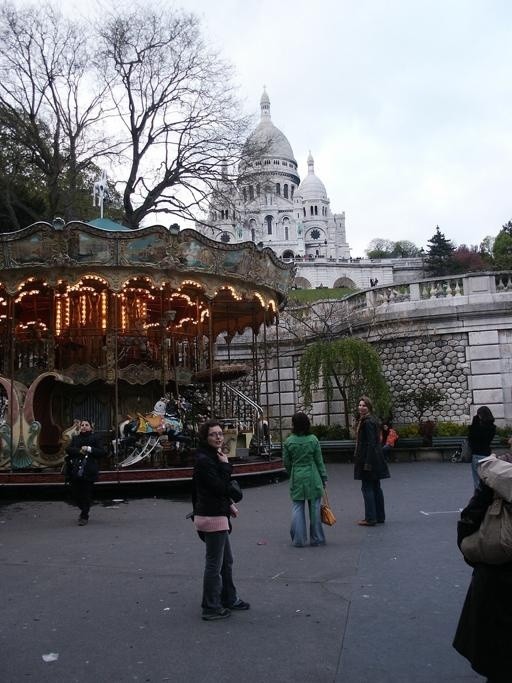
xmin=369 ymin=277 xmax=376 ymax=293
xmin=379 ymin=423 xmax=400 ymax=460
xmin=61 ymin=416 xmax=110 ymax=526
xmin=187 ymin=420 xmax=251 ymax=622
xmin=449 ymin=403 xmax=512 ymax=682
xmin=276 ymin=252 xmax=374 ymax=265
xmin=282 ymin=412 xmax=331 ymax=547
xmin=353 ymin=396 xmax=392 ymax=526
xmin=373 ymin=277 xmax=379 ymax=285
xmin=465 ymin=405 xmax=498 ymax=496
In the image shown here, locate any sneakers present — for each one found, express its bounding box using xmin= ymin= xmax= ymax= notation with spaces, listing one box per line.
xmin=225 ymin=598 xmax=251 ymax=610
xmin=357 ymin=516 xmax=385 ymax=526
xmin=199 ymin=605 xmax=233 ymax=621
xmin=78 ymin=517 xmax=89 ymax=526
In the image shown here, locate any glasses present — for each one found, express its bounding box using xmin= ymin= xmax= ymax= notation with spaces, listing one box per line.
xmin=208 ymin=430 xmax=224 ymax=436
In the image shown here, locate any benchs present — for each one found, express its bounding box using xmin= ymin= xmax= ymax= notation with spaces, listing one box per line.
xmin=272 ymin=435 xmax=508 ymax=463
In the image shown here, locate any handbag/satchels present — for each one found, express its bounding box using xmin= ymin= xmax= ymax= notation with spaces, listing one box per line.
xmin=61 ymin=456 xmax=88 ymax=483
xmin=321 ymin=502 xmax=337 ymax=526
xmin=457 ymin=453 xmax=512 ymax=568
